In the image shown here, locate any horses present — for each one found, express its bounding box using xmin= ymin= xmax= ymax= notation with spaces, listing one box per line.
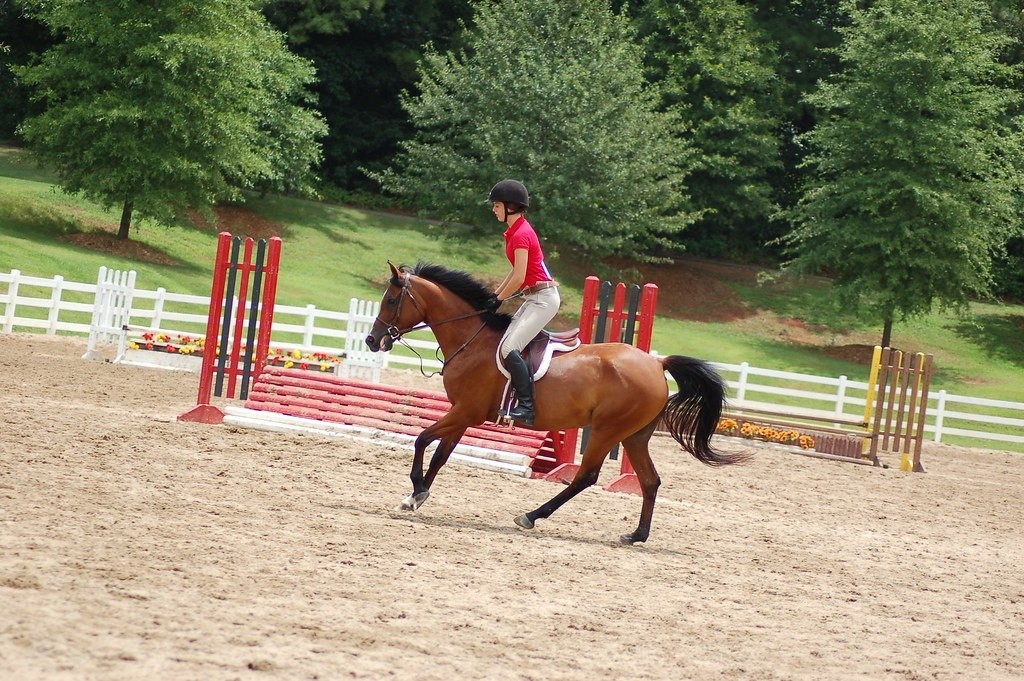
xmin=365 ymin=259 xmax=757 ymax=548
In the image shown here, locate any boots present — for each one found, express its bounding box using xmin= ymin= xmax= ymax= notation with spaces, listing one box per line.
xmin=497 ymin=349 xmax=535 ymax=426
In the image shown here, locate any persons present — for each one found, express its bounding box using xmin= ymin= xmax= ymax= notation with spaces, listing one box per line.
xmin=488 ymin=179 xmax=560 ymax=427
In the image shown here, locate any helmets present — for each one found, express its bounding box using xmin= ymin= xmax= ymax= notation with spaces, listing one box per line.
xmin=484 ymin=180 xmax=529 ymax=210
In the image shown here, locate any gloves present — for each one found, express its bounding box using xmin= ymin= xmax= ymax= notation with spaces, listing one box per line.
xmin=489 ymin=293 xmax=504 ymax=313
